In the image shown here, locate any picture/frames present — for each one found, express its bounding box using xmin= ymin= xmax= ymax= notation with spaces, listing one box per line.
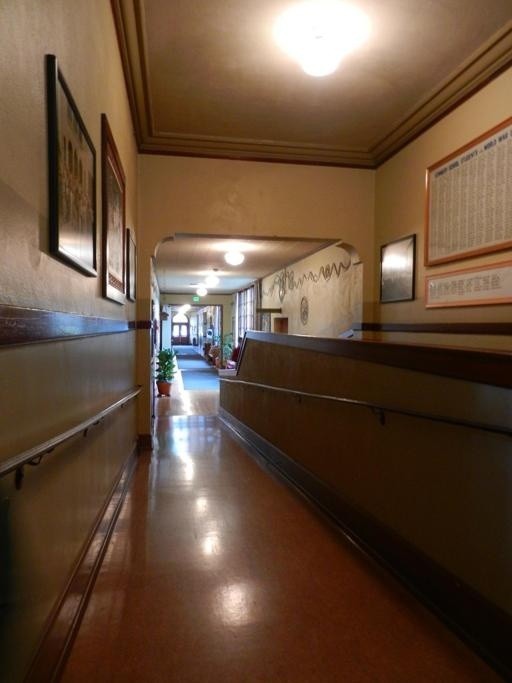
xmin=425 ymin=115 xmax=512 ymax=308
xmin=44 ymin=53 xmax=139 ymax=307
xmin=379 ymin=233 xmax=415 ymax=303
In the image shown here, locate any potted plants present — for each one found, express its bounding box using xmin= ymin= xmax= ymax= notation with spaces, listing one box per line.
xmin=155 ymin=346 xmax=178 ymax=396
